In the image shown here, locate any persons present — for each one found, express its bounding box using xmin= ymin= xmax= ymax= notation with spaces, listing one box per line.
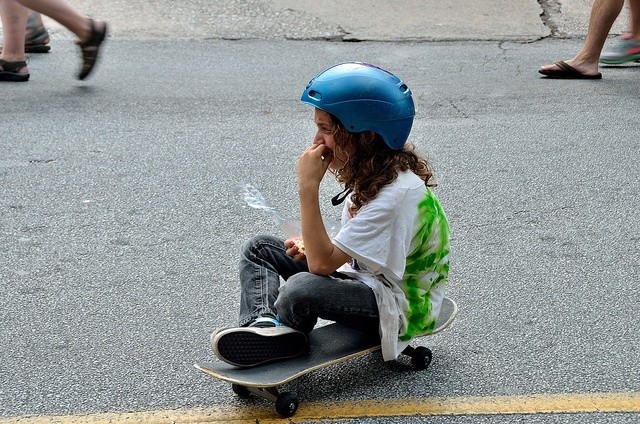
xmin=0 ymin=7 xmax=52 ymax=53
xmin=538 ymin=0 xmax=640 ymax=79
xmin=210 ymin=61 xmax=451 ymax=368
xmin=0 ymin=0 xmax=107 ymax=81
xmin=599 ymin=0 xmax=640 ymax=64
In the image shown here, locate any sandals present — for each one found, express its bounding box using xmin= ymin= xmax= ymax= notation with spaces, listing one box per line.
xmin=0 ymin=59 xmax=30 ymax=82
xmin=0 ymin=25 xmax=51 ymax=52
xmin=75 ymin=18 xmax=107 ymax=80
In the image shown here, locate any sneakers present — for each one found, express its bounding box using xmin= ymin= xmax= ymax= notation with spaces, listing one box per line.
xmin=599 ymin=34 xmax=640 ymax=64
xmin=210 ymin=314 xmax=307 ymax=367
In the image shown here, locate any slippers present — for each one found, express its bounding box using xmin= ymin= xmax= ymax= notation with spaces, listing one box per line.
xmin=538 ymin=61 xmax=602 ymax=79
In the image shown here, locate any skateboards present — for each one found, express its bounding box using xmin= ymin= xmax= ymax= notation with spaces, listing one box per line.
xmin=194 ymin=296 xmax=458 ymax=417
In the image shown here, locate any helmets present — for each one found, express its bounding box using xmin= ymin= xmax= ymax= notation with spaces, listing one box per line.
xmin=301 ymin=61 xmax=415 ymax=149
